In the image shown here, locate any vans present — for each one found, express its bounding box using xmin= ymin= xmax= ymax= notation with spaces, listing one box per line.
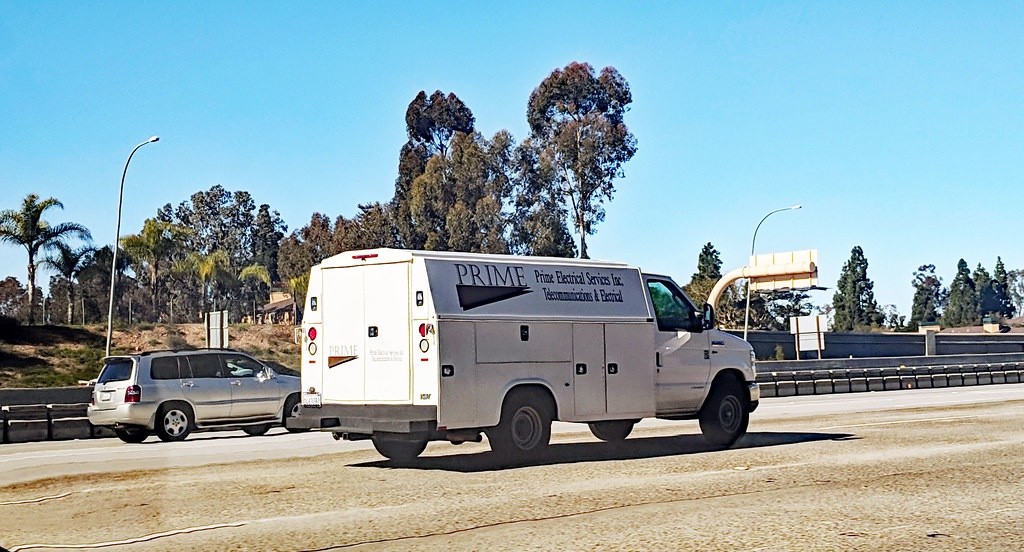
xmin=85 ymin=351 xmax=312 ymax=444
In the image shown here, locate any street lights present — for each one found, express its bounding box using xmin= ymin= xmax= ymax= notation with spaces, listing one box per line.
xmin=743 ymin=205 xmax=802 ymax=340
xmin=105 ymin=135 xmax=160 ymax=354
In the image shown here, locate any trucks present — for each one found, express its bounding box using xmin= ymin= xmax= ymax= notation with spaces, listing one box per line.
xmin=296 ymin=246 xmax=761 ymax=461
